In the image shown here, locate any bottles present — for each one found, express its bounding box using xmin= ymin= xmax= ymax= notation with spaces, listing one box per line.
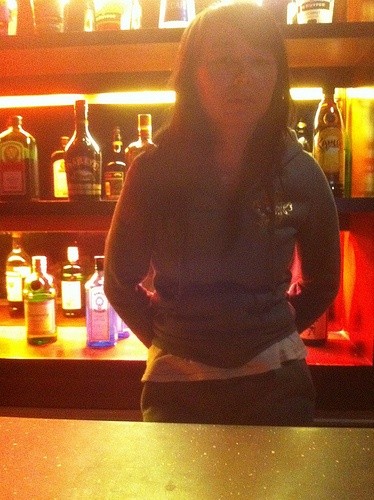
xmin=0 ymin=115 xmax=39 ymax=202
xmin=86 ymin=256 xmax=131 ymax=348
xmin=61 ymin=241 xmax=88 ymax=318
xmin=64 ymin=100 xmax=102 ymax=203
xmin=5 ymin=232 xmax=32 ymax=319
xmin=300 ymin=310 xmax=328 ymax=346
xmin=313 ymin=86 xmax=345 ymax=199
xmin=125 ymin=114 xmax=158 ymax=172
xmin=0 ymin=0 xmax=142 ymax=36
xmin=104 ymin=127 xmax=127 ymax=200
xmin=158 ymin=0 xmax=196 ymax=29
xmin=296 ymin=121 xmax=311 ymax=153
xmin=51 ymin=136 xmax=70 ymax=199
xmin=23 ymin=255 xmax=57 ymax=346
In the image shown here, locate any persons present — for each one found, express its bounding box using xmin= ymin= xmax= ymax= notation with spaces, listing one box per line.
xmin=103 ymin=1 xmax=341 ymax=426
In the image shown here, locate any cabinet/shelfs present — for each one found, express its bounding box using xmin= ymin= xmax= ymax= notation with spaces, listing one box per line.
xmin=0 ymin=0 xmax=374 ymax=391
